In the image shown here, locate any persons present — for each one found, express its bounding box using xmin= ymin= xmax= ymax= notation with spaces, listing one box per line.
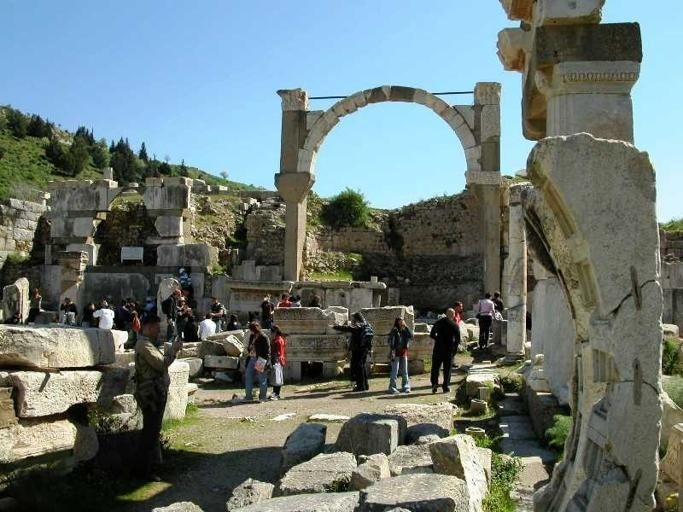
xmin=242 ymin=321 xmax=271 ymax=403
xmin=387 ymin=315 xmax=414 ymax=394
xmin=491 ymin=291 xmax=503 ymax=312
xmin=452 ymin=301 xmax=463 ymax=368
xmin=61 ymin=268 xmax=322 ymax=342
xmin=268 ymin=326 xmax=289 ymax=401
xmin=430 ymin=308 xmax=460 ymax=393
xmin=134 ymin=314 xmax=183 ymax=482
xmin=24 ymin=288 xmax=42 ymax=324
xmin=478 ymin=293 xmax=495 ymax=348
xmin=328 ymin=312 xmax=374 ymax=391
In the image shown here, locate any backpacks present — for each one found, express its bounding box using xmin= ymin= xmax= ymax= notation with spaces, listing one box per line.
xmin=360 ymin=327 xmax=375 ymax=348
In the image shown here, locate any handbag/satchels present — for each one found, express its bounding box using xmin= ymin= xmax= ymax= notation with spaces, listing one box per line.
xmin=134 ymin=379 xmax=166 ymax=411
xmin=269 ymin=361 xmax=284 ymax=386
